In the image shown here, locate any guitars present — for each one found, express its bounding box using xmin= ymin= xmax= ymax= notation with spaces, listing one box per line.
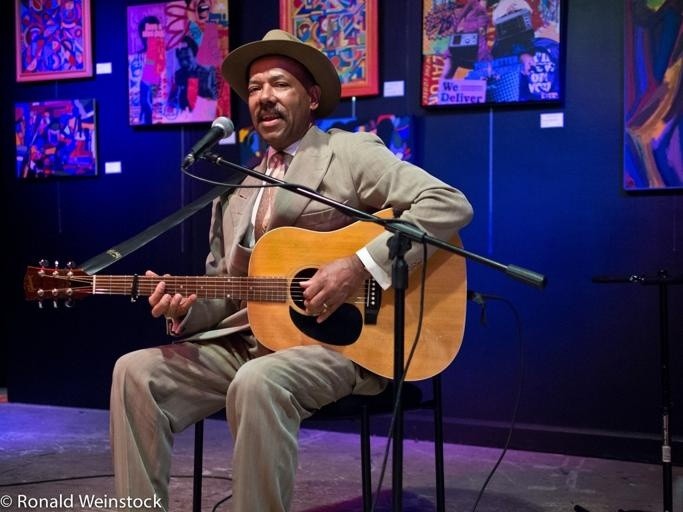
xmin=24 ymin=205 xmax=467 ymax=382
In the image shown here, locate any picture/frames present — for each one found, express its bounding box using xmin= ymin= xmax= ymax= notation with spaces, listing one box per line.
xmin=279 ymin=0 xmax=379 ymax=97
xmin=14 ymin=0 xmax=93 ymax=83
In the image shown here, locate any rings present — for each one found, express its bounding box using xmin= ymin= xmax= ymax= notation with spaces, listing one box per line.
xmin=321 ymin=302 xmax=328 ymax=315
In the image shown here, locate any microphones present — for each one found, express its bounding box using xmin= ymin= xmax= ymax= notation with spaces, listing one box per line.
xmin=182 ymin=116 xmax=235 ymax=170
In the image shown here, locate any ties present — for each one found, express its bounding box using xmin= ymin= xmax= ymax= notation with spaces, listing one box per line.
xmin=255 ymin=151 xmax=288 ymax=244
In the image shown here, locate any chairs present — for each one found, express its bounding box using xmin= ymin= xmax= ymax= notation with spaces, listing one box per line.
xmin=193 ymin=371 xmax=445 ymax=512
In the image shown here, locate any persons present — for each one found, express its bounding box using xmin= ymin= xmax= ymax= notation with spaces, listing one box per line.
xmin=110 ymin=29 xmax=473 ymax=512
xmin=166 ymin=35 xmax=216 ymax=108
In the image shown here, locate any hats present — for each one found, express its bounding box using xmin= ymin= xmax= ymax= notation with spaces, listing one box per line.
xmin=221 ymin=29 xmax=341 ymax=118
